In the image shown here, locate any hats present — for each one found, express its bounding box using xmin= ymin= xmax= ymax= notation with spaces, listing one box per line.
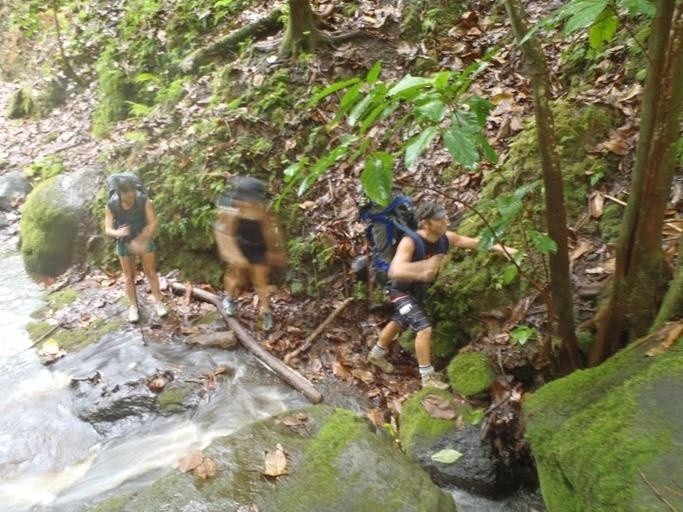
xmin=418 ymin=202 xmax=449 ymax=220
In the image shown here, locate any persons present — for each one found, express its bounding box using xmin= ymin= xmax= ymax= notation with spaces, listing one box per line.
xmin=214 ymin=175 xmax=286 ymax=331
xmin=366 ymin=203 xmax=528 ymax=392
xmin=104 ymin=173 xmax=167 ymax=324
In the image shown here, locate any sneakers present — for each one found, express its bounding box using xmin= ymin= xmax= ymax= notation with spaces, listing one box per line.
xmin=263 ymin=312 xmax=273 ymax=330
xmin=223 ymin=297 xmax=235 ymax=316
xmin=154 ymin=301 xmax=168 ymax=316
xmin=129 ymin=305 xmax=139 ymax=321
xmin=421 ymin=372 xmax=451 ymax=390
xmin=367 ymin=352 xmax=394 ymax=373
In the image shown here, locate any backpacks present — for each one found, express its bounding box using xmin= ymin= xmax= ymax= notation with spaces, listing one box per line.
xmin=107 ymin=174 xmax=142 ymax=210
xmin=359 ymin=186 xmax=423 ymax=284
